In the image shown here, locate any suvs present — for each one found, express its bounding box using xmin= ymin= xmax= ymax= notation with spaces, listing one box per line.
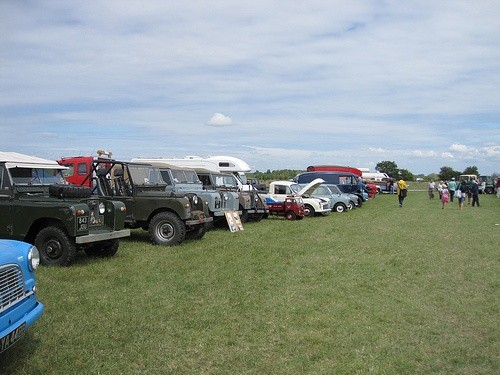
xmin=56 ymin=150 xmax=269 ymax=247
xmin=0 ymin=239 xmax=44 ymax=357
xmin=0 ymin=152 xmax=130 ymax=269
xmin=459 ymin=175 xmax=493 ymax=194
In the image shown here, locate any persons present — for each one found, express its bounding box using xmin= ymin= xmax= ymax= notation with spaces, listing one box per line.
xmin=437 ymin=181 xmax=443 ymax=199
xmin=439 ymin=184 xmax=449 ymax=210
xmin=396 ymin=176 xmax=407 ymax=207
xmin=428 ymin=179 xmax=436 ymax=200
xmin=388 ymin=179 xmax=394 ymax=191
xmin=493 ymin=178 xmax=500 ymax=198
xmin=470 ymin=180 xmax=480 ymax=207
xmin=442 ymin=178 xmax=472 ymax=204
xmin=457 ymin=179 xmax=466 ymax=211
xmin=481 ymin=179 xmax=487 ymax=196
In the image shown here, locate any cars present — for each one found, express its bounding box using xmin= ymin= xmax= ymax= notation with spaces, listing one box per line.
xmin=255 ymin=178 xmax=351 ymax=221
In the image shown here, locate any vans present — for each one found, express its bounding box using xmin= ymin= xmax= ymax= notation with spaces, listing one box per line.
xmin=293 ymin=165 xmax=387 ymax=202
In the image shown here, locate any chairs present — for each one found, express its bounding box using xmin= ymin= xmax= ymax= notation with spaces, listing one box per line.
xmin=114 ymin=169 xmax=131 ymax=196
xmin=97 ymin=168 xmax=113 ymax=196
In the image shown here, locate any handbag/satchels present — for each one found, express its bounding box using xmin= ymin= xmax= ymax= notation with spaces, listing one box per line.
xmin=453 ymin=184 xmax=462 ymax=198
xmin=400 ymin=188 xmax=408 ymax=198
